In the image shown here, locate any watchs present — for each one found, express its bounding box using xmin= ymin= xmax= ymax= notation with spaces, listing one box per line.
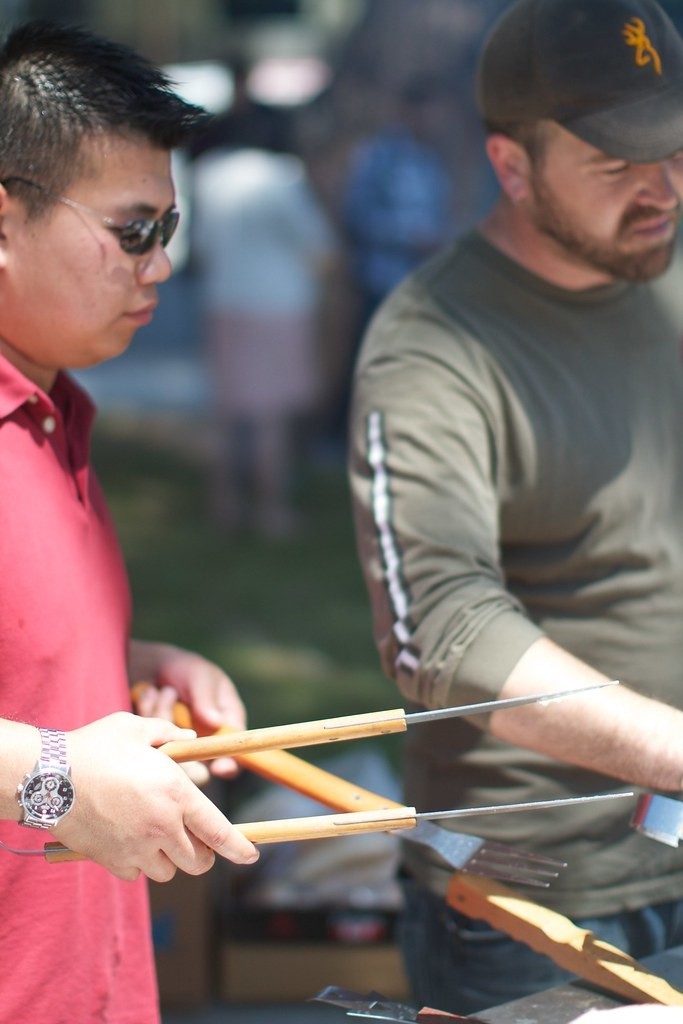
xmin=19 ymin=724 xmax=76 ymax=828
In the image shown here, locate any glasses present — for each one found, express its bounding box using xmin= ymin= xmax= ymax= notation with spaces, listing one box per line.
xmin=1 ymin=176 xmax=181 ymax=254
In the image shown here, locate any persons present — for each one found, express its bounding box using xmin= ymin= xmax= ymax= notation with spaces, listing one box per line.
xmin=187 ymin=74 xmax=455 ymax=560
xmin=348 ymin=1 xmax=683 ymax=1009
xmin=0 ymin=20 xmax=265 ymax=1023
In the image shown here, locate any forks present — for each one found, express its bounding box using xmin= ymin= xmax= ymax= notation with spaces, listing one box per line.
xmin=129 ymin=677 xmax=569 ymax=888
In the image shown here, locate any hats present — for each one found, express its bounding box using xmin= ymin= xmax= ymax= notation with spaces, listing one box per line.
xmin=475 ymin=0 xmax=682 ymax=165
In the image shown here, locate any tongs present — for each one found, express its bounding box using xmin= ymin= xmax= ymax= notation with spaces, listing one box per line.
xmin=0 ymin=680 xmax=635 ymax=862
